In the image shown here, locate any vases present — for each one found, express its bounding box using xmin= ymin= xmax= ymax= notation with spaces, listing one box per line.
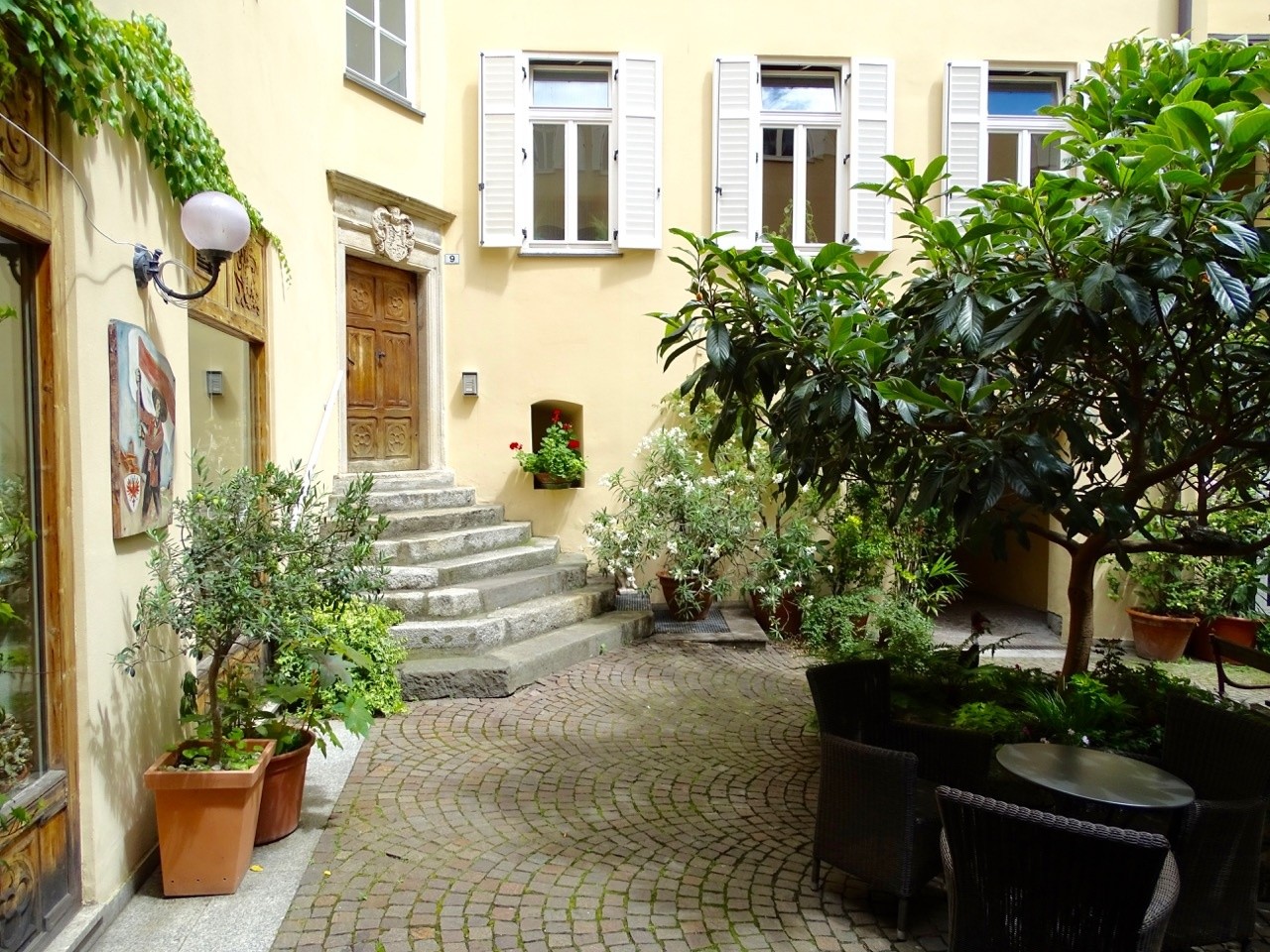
xmin=536 ymin=473 xmax=571 ymax=489
xmin=656 ymin=571 xmax=719 ymax=620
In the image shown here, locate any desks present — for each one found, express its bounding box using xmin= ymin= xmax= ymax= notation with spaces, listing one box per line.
xmin=996 ymin=743 xmax=1195 ymax=829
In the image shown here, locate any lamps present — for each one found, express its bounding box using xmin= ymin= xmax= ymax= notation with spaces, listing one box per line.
xmin=134 ymin=191 xmax=251 ymax=300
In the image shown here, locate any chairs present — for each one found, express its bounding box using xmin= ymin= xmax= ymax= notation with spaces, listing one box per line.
xmin=935 ymin=785 xmax=1182 ymax=952
xmin=1125 ymin=689 xmax=1270 ymax=952
xmin=807 ymin=660 xmax=991 ymax=941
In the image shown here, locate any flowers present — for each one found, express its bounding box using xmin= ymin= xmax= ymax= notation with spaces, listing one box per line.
xmin=510 ymin=410 xmax=589 ymax=484
xmin=584 ymin=401 xmax=835 ymax=643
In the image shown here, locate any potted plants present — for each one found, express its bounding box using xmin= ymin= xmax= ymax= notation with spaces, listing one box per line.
xmin=659 ymin=377 xmax=870 ymax=638
xmin=107 ymin=448 xmax=408 ymax=896
xmin=1097 ymin=461 xmax=1270 ymax=665
